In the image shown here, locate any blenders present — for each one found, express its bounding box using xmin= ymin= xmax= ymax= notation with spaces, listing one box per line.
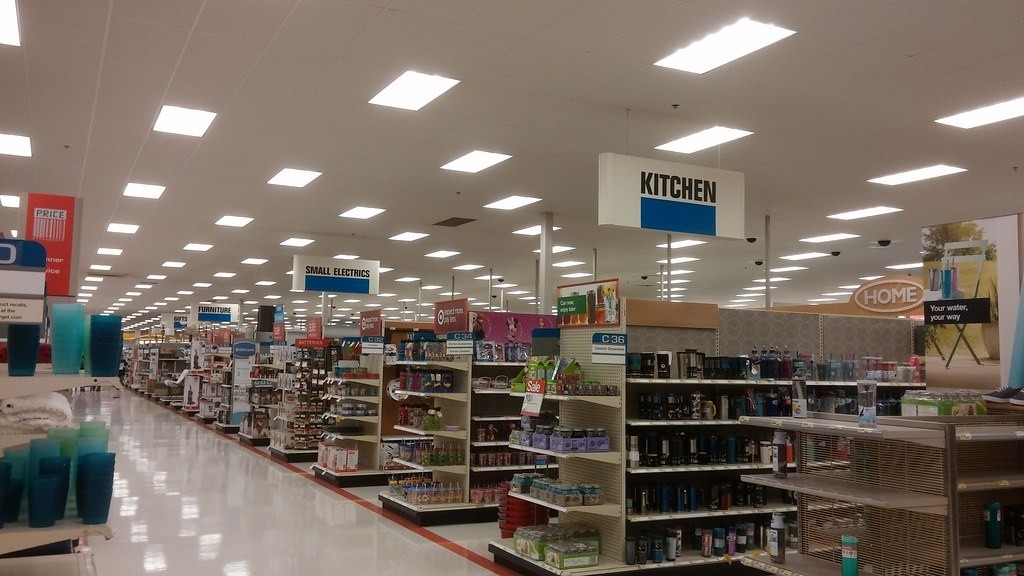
xmin=676 ymin=348 xmax=706 ymax=378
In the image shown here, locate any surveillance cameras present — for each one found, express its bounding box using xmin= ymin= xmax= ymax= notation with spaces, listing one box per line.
xmin=878 ymin=241 xmax=891 ymax=246
xmin=641 ymin=276 xmax=647 ymax=280
xmin=755 ymin=261 xmax=763 ymax=265
xmin=405 ymin=307 xmax=407 ymax=309
xmin=353 ymin=322 xmax=354 ymax=323
xmin=332 ymin=306 xmax=336 ymax=308
xmin=498 ymin=279 xmax=504 ymax=282
xmin=492 ymin=296 xmax=496 ymax=298
xmin=831 ymin=251 xmax=840 ymax=256
xmin=351 ymin=314 xmax=354 ymax=315
xmin=747 ymin=238 xmax=756 ymax=243
xmin=293 ymin=313 xmax=296 ymax=314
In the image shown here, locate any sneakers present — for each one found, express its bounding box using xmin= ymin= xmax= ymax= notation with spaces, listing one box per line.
xmin=982 ymin=386 xmax=1023 ymax=403
xmin=1009 ymin=387 xmax=1024 ymax=405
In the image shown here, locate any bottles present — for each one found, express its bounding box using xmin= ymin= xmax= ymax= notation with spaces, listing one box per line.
xmin=605 ymin=288 xmax=617 ymax=323
xmin=899 ymin=389 xmax=986 ymax=418
xmin=625 ymin=432 xmax=641 ymax=467
xmin=398 ymin=365 xmax=453 ymax=393
xmin=772 ymin=429 xmax=794 ymax=478
xmin=745 ymin=345 xmax=806 ymax=380
xmin=637 ymin=387 xmax=684 ymax=421
xmin=769 ymin=510 xmax=787 ymax=564
xmin=735 ymin=386 xmax=792 ymax=421
xmin=557 ymin=290 xmax=596 ymax=324
xmin=626 ymin=476 xmax=799 ymax=515
xmin=399 ymin=403 xmax=443 ymax=432
xmin=503 ymin=424 xmax=609 ymax=559
xmin=398 ymin=337 xmax=453 ymax=360
xmin=792 ymin=368 xmax=808 ymax=418
xmin=327 ymin=385 xmax=377 ymax=415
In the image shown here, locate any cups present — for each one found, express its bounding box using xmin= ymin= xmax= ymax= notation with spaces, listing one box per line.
xmin=661 ymin=434 xmax=772 ymax=464
xmin=83 ymin=314 xmax=124 ymax=377
xmin=471 ymin=451 xmax=533 ymax=466
xmin=982 ymin=502 xmax=1001 ymax=549
xmin=657 ymin=350 xmax=673 ymax=379
xmin=691 ymin=390 xmax=701 ymax=420
xmin=962 ymin=564 xmax=1017 ymax=576
xmin=399 ymin=440 xmax=466 ymax=467
xmin=700 ymin=399 xmax=716 ymax=420
xmin=817 ymin=362 xmax=827 ymax=382
xmin=877 ymin=401 xmax=901 ymax=416
xmin=470 ymin=484 xmax=500 ymax=504
xmin=0 ymin=420 xmax=115 ymax=527
xmin=840 ymin=535 xmax=858 ymax=576
xmin=1004 ymin=504 xmax=1024 ymax=546
xmin=389 ymin=477 xmax=462 ymax=506
xmin=827 ymin=357 xmax=921 ymax=382
xmin=477 ymin=423 xmax=515 ymax=443
xmin=716 ymin=394 xmax=729 ymax=420
xmin=7 ymin=324 xmax=40 ymax=377
xmin=857 ymin=379 xmax=876 ymax=428
xmin=696 ymin=522 xmax=766 ymax=557
xmin=645 ymin=452 xmax=658 ymax=467
xmin=624 ymin=524 xmax=682 ymax=564
xmin=50 ymin=302 xmax=86 ymax=375
xmin=807 ymin=393 xmax=856 ymax=414
xmin=927 ymin=267 xmax=958 ymax=298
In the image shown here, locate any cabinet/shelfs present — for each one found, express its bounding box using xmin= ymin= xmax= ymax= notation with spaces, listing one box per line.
xmin=0 ymin=364 xmax=125 ymax=576
xmin=377 ymin=359 xmax=481 ymax=527
xmin=195 ymin=353 xmax=217 ymax=425
xmin=267 ymin=360 xmax=318 ymax=463
xmin=311 ymin=353 xmax=385 ymax=489
xmin=468 ymin=310 xmax=559 ymax=524
xmin=626 ymin=378 xmax=926 ymax=576
xmin=211 ymin=353 xmax=240 ymax=434
xmin=379 ymin=321 xmax=433 ymax=486
xmin=488 ymin=391 xmax=641 ymax=576
xmin=955 ymin=421 xmax=1024 ymax=568
xmin=123 ymin=342 xmax=199 ymax=417
xmin=737 ymin=412 xmax=953 ymax=576
xmin=236 ymin=363 xmax=285 ymax=447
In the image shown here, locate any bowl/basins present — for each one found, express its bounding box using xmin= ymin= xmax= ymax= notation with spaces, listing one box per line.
xmin=341 ymin=367 xmax=380 ymax=379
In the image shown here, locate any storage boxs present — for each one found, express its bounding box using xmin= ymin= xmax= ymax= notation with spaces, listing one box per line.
xmin=569 ymin=536 xmax=601 ymax=554
xmin=380 ymin=442 xmax=416 ymax=471
xmin=318 ymin=442 xmax=335 ymax=467
xmin=546 ymin=382 xmax=621 ymax=396
xmin=535 ymin=361 xmax=546 ymax=379
xmin=475 ymin=341 xmax=496 ymax=362
xmin=510 ymin=368 xmax=526 ymax=393
xmin=495 ymin=342 xmax=505 ymax=362
xmin=897 ymin=397 xmax=987 ymax=416
xmin=154 ymin=388 xmax=169 ymax=396
xmin=327 ymin=446 xmax=347 ymax=473
xmin=184 ymin=340 xmax=296 ymax=450
xmin=528 ymin=360 xmax=538 ymax=380
xmin=546 ymin=361 xmax=555 ymax=380
xmin=543 ymin=546 xmax=599 ymax=570
xmin=344 ymin=448 xmax=358 ymax=472
xmin=145 ymin=379 xmax=157 ymax=393
xmin=513 ymin=533 xmax=548 ymax=562
xmin=16 ymin=191 xmax=84 ymax=297
